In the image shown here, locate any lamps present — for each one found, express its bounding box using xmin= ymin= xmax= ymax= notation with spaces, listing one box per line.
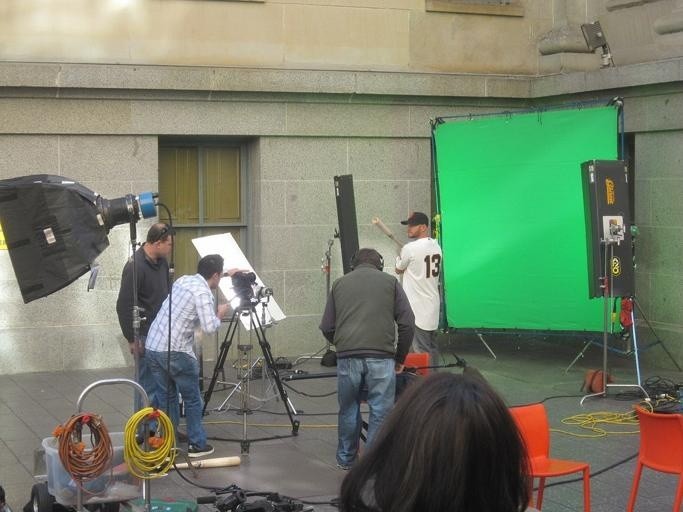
xmin=581 ymin=18 xmax=615 ymax=69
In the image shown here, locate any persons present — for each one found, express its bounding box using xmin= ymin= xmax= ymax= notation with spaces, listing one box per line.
xmin=116 ymin=222 xmax=188 ymax=443
xmin=318 ymin=248 xmax=415 ymax=470
xmin=337 ymin=373 xmax=534 ymax=511
xmin=145 ymin=255 xmax=230 ymax=458
xmin=394 ymin=213 xmax=443 ymax=371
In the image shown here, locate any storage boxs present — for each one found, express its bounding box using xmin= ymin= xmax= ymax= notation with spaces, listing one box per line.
xmin=40 ymin=432 xmax=148 ymax=505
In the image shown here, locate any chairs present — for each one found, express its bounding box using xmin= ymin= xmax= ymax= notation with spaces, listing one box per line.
xmin=627 ymin=401 xmax=682 ymax=510
xmin=405 ymin=352 xmax=428 ymax=376
xmin=508 ymin=400 xmax=593 ymax=511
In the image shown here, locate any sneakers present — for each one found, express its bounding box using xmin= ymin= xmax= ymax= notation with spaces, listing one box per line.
xmin=187 ymin=445 xmax=214 ymax=458
xmin=166 ymin=453 xmax=180 ymax=461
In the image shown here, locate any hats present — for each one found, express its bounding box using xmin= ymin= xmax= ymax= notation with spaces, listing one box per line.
xmin=401 ymin=212 xmax=428 ymax=226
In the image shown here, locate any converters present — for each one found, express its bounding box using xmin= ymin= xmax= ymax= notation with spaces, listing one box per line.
xmin=268 ymin=361 xmax=292 ymax=369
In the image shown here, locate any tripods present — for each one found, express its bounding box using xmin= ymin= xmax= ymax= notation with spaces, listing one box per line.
xmin=200 ymin=305 xmax=300 ymax=456
